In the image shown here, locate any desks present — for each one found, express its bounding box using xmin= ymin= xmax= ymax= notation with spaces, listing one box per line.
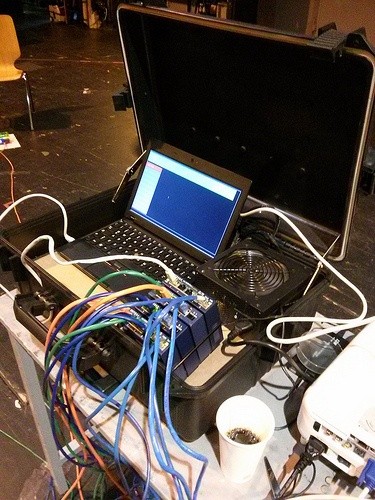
xmin=0 ymin=289 xmax=356 ymax=500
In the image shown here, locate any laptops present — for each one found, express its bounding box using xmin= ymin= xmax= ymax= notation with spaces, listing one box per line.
xmin=57 ymin=137 xmax=253 ymax=310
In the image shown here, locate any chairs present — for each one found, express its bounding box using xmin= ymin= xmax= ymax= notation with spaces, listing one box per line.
xmin=0 ymin=15 xmax=36 ymax=131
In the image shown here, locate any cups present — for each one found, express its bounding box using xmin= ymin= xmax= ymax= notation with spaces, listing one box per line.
xmin=212 ymin=395 xmax=278 ymax=481
